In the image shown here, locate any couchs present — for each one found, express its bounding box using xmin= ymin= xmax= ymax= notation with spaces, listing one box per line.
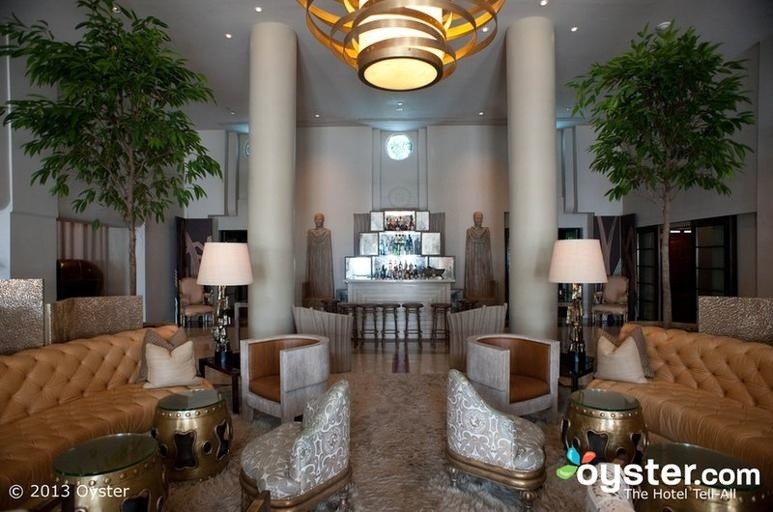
xmin=1 ymin=323 xmax=214 ymax=512
xmin=586 ymin=322 xmax=773 ymax=493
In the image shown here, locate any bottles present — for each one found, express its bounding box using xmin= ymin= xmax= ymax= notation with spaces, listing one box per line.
xmin=380 ymin=234 xmax=420 ymax=255
xmin=385 ymin=215 xmax=415 ymax=230
xmin=375 ymin=261 xmax=417 ymax=280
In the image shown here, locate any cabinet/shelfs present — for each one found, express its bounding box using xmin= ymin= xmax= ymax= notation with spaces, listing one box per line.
xmin=504 ymin=212 xmax=593 ymax=333
xmin=343 ymin=208 xmax=456 ymax=339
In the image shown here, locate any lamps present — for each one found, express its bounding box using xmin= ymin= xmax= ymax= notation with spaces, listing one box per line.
xmin=296 ymin=0 xmax=506 ymax=92
xmin=195 ymin=241 xmax=254 ymax=371
xmin=546 ymin=238 xmax=609 ymax=363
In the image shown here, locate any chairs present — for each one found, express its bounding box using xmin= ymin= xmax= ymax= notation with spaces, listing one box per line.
xmin=592 ymin=275 xmax=630 ymax=327
xmin=586 ymin=462 xmax=636 ymax=512
xmin=178 ymin=276 xmax=215 ymax=337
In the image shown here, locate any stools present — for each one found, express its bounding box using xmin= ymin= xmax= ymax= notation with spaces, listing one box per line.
xmin=321 ymin=299 xmax=338 ymax=313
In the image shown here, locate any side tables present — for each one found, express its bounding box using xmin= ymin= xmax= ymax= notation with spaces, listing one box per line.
xmin=199 ymin=352 xmax=240 ymax=415
xmin=560 ymin=352 xmax=595 ymax=393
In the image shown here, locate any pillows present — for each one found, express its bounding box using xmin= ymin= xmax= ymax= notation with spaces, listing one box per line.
xmin=143 ymin=341 xmax=198 ymax=390
xmin=140 ymin=332 xmax=198 ymax=380
xmin=595 ymin=328 xmax=654 ymax=378
xmin=596 ymin=336 xmax=649 ymax=384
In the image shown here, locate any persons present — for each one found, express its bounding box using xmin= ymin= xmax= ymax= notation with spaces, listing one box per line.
xmin=464 ymin=211 xmax=495 ymax=306
xmin=306 ymin=213 xmax=337 ymax=301
